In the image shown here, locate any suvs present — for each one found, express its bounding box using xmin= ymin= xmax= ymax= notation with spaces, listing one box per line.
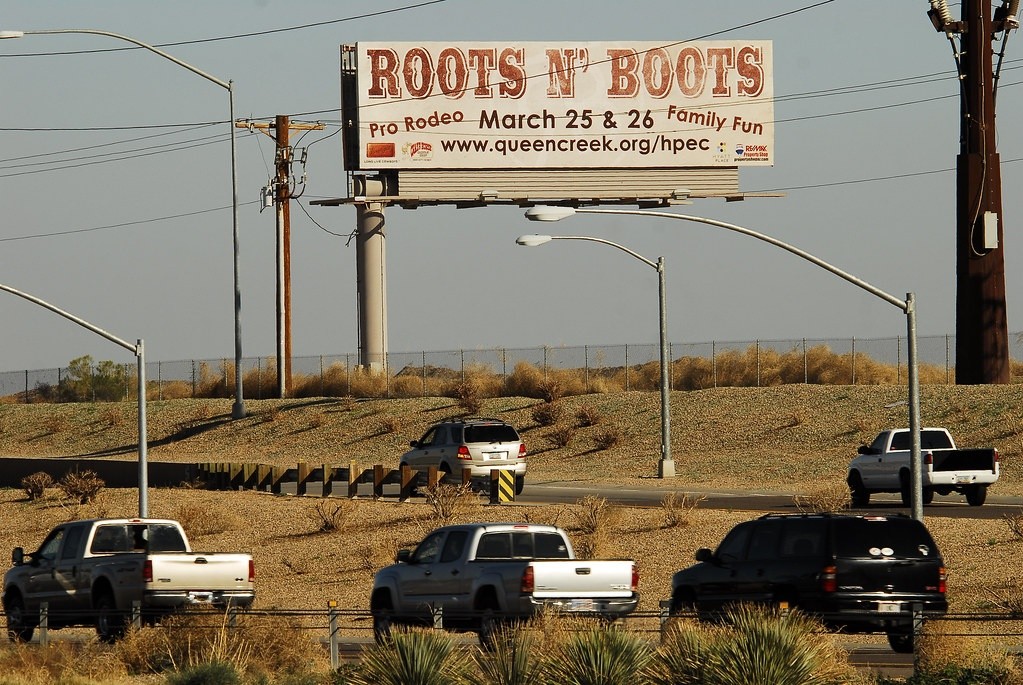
xmin=659 ymin=508 xmax=951 ymax=653
xmin=400 ymin=413 xmax=526 ymax=497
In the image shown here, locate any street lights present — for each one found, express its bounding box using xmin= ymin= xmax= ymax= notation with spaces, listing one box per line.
xmin=0 ymin=29 xmax=247 ymax=420
xmin=0 ymin=282 xmax=149 ymax=516
xmin=516 ymin=234 xmax=678 ymax=479
xmin=525 ymin=200 xmax=925 ymax=523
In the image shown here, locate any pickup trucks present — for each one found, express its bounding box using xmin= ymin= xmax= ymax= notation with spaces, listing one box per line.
xmin=1 ymin=513 xmax=258 ymax=648
xmin=847 ymin=428 xmax=1002 ymax=507
xmin=370 ymin=522 xmax=643 ymax=652
